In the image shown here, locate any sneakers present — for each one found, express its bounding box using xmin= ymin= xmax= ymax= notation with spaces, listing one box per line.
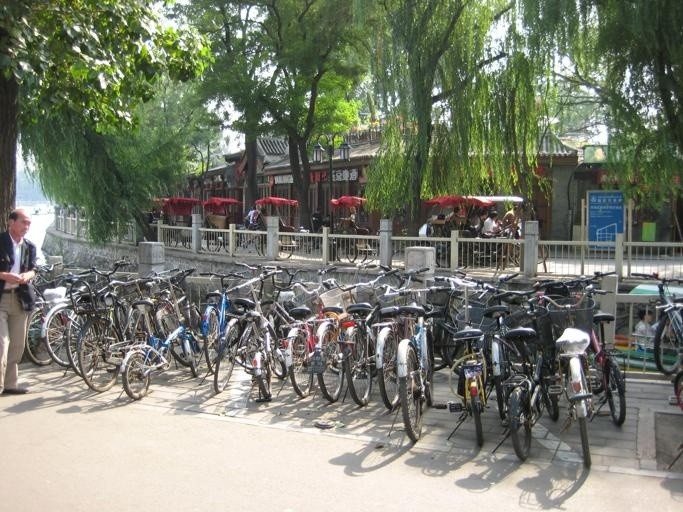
xmin=1 ymin=389 xmax=27 ymax=395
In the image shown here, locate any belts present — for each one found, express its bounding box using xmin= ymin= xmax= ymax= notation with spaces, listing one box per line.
xmin=1 ymin=288 xmax=20 ymax=294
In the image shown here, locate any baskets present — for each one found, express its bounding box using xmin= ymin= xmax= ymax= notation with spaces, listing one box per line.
xmin=547 ymin=299 xmax=594 ymax=337
xmin=319 ymin=287 xmax=357 ymax=321
xmin=504 ymin=306 xmax=556 ymax=356
xmin=291 ymin=287 xmax=322 ymax=316
xmin=455 ymin=307 xmax=495 ymax=334
xmin=159 ymin=275 xmax=187 ymax=293
xmin=374 ymin=283 xmax=408 ymax=306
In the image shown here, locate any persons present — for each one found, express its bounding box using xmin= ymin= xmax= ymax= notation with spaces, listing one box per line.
xmin=340 ymin=206 xmax=357 ymax=227
xmin=0 ymin=209 xmax=40 ymax=396
xmin=322 ymin=215 xmax=330 ymax=227
xmin=313 ymin=207 xmax=324 ymax=233
xmin=214 ymin=202 xmax=225 ymax=216
xmin=634 ymin=308 xmax=667 ymax=354
xmin=245 ymin=204 xmax=263 ymax=230
xmin=431 ymin=206 xmax=520 ymax=238
